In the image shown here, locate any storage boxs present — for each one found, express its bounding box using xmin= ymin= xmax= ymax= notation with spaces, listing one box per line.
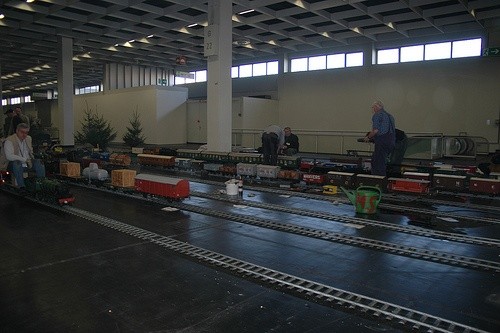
xmin=59 ymin=161 xmax=80 ymax=177
xmin=111 ymin=170 xmax=136 ymax=187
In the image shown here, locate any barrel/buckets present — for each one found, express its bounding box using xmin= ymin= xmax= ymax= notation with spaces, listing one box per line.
xmin=225 ymin=179 xmax=239 ymax=195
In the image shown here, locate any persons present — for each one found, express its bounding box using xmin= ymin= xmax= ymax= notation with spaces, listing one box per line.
xmin=367 ymin=127 xmax=407 ymax=177
xmin=1 ymin=123 xmax=47 ymax=192
xmin=260 ymin=125 xmax=285 ymax=166
xmin=364 ymin=99 xmax=396 ymax=176
xmin=1 ymin=108 xmax=30 ymax=138
xmin=280 ymin=127 xmax=299 ymax=155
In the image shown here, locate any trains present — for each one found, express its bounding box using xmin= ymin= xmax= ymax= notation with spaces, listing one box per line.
xmin=50 ymin=145 xmax=130 ymax=166
xmin=136 ymin=148 xmax=500 ymax=196
xmin=59 ymin=160 xmax=192 ymax=199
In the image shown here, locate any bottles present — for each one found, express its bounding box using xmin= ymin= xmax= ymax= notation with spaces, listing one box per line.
xmin=238 ymin=176 xmax=243 ymax=197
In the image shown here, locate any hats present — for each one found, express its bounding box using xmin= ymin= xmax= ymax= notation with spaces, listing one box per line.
xmin=4 ymin=109 xmax=13 ymax=114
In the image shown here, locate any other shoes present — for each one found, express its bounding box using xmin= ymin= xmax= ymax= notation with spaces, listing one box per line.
xmin=19 ymin=185 xmax=26 ymax=193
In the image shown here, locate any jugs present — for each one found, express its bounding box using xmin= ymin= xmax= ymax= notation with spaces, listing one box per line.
xmin=339 ymin=185 xmax=382 ymax=214
